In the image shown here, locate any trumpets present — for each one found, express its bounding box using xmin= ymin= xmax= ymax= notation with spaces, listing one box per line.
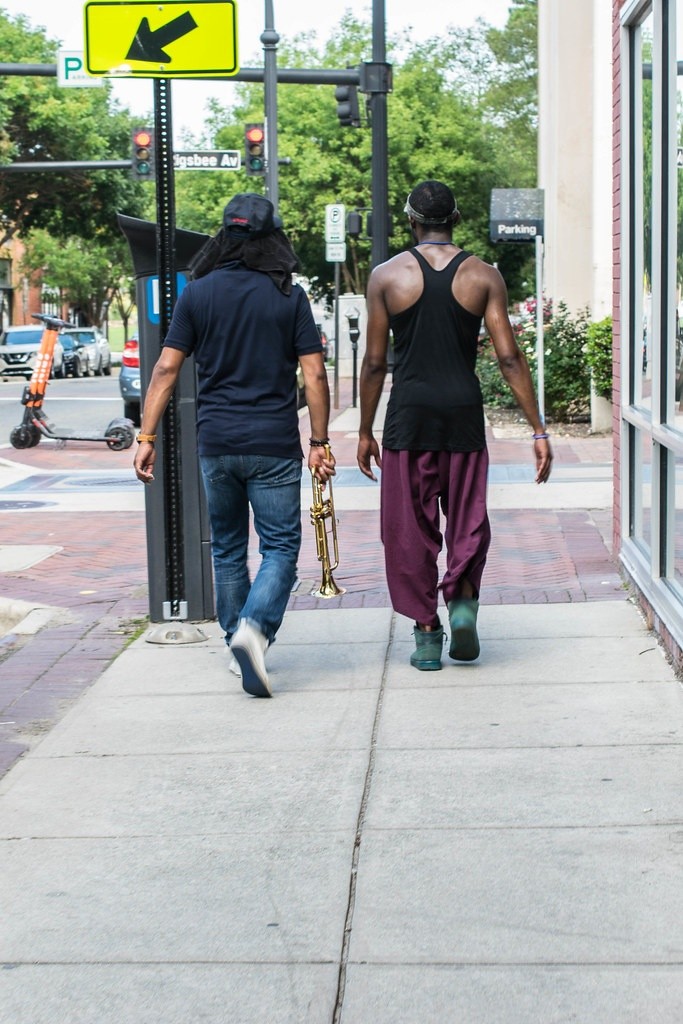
xmin=308 ymin=442 xmax=347 ymax=599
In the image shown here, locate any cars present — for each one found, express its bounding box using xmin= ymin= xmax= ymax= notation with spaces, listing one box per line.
xmin=120 ymin=328 xmax=140 ymax=429
xmin=296 ymin=365 xmax=306 ymax=408
xmin=59 ymin=333 xmax=92 ymax=378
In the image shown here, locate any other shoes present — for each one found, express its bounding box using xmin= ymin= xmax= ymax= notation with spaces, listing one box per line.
xmin=229 ymin=656 xmax=242 ymax=678
xmin=447 ymin=598 xmax=480 ymax=661
xmin=229 ymin=617 xmax=272 ymax=697
xmin=409 ymin=625 xmax=447 ymax=669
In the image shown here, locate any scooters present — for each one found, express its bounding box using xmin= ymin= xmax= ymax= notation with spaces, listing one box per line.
xmin=10 ymin=313 xmax=136 ymax=450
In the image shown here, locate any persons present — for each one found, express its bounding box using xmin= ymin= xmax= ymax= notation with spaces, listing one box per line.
xmin=357 ymin=181 xmax=552 ymax=671
xmin=133 ymin=194 xmax=336 ymax=698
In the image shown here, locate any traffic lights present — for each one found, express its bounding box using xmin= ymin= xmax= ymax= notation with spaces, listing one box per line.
xmin=245 ymin=123 xmax=267 ymax=175
xmin=131 ymin=128 xmax=155 ymax=182
xmin=335 ymin=80 xmax=361 ymax=128
xmin=347 ymin=212 xmax=362 ymax=236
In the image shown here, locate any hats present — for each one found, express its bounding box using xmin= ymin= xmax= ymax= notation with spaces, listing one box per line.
xmin=403 ymin=193 xmax=457 ymax=224
xmin=222 ymin=193 xmax=282 ymax=239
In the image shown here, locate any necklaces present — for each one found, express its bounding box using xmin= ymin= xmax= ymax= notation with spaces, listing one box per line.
xmin=418 ymin=242 xmax=454 ymax=245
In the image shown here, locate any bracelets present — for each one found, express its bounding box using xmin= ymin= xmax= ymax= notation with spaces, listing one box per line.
xmin=534 ymin=435 xmax=548 ymax=439
xmin=136 ymin=431 xmax=157 ymax=444
xmin=310 ymin=438 xmax=329 ymax=446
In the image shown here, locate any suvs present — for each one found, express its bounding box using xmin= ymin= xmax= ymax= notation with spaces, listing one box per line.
xmin=0 ymin=324 xmax=66 ymax=380
xmin=62 ymin=326 xmax=112 ymax=377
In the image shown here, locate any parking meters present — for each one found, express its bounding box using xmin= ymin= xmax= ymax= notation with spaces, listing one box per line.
xmin=344 ymin=306 xmax=360 ymax=408
